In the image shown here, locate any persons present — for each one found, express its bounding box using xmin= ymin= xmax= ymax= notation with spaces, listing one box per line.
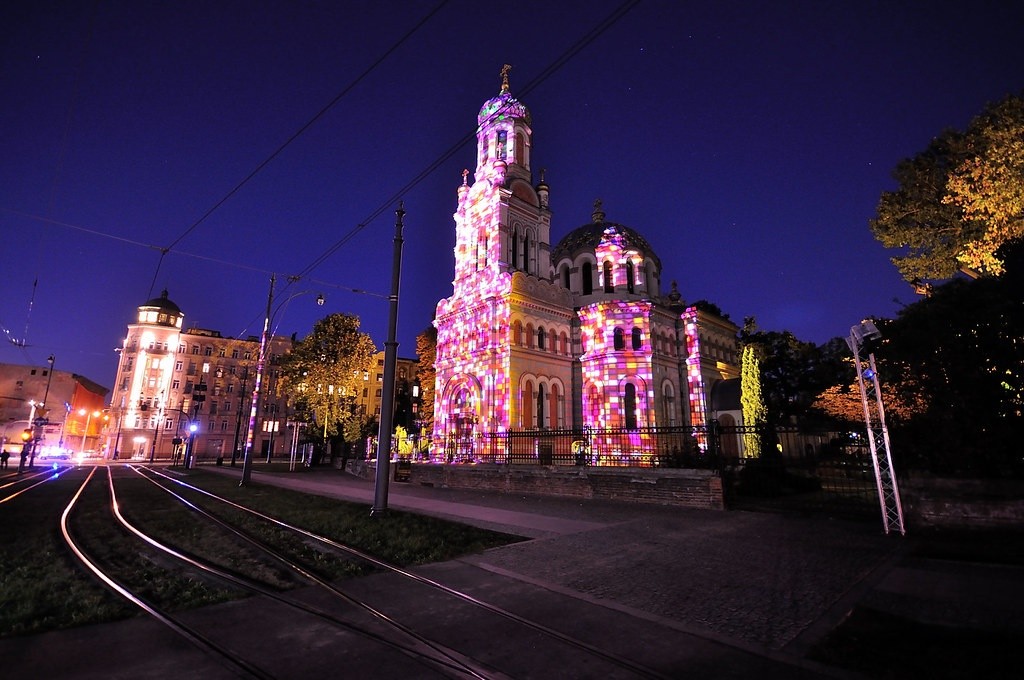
xmin=0 ymin=449 xmax=10 ymax=470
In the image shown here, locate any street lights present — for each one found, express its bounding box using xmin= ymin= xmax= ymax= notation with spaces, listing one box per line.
xmin=78 ymin=410 xmax=101 ymax=462
xmin=26 ymin=355 xmax=56 ymax=469
xmin=239 ymin=290 xmax=326 ymax=484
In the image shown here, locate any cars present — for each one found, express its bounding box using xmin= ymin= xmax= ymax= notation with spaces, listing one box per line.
xmin=39 ymin=447 xmax=70 ymax=461
xmin=82 ymin=450 xmax=97 ymax=459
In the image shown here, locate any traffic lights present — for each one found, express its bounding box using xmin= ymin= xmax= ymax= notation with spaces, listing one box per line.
xmin=21 ymin=429 xmax=31 ymax=443
xmin=190 ymin=423 xmax=198 ymax=433
